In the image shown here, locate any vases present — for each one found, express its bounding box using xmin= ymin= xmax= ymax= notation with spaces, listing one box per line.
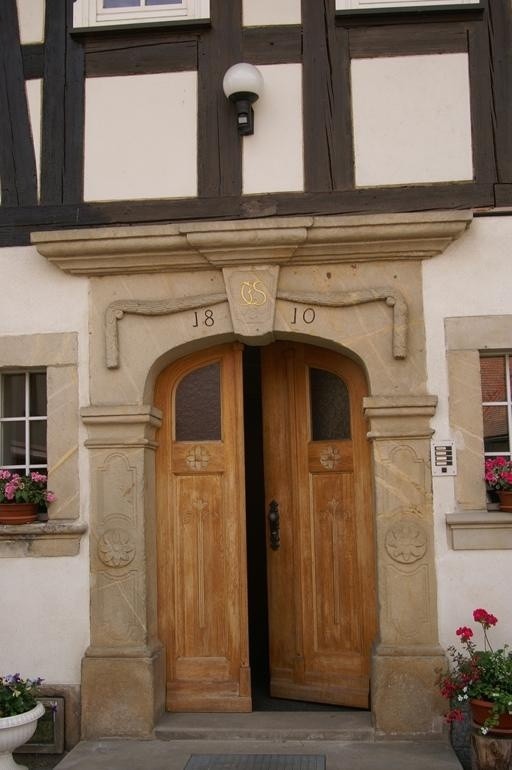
xmin=471 ymin=700 xmax=511 ymax=734
xmin=0 ymin=502 xmax=39 ymax=525
xmin=496 ymin=491 xmax=512 ymax=512
xmin=0 ymin=701 xmax=45 ymax=769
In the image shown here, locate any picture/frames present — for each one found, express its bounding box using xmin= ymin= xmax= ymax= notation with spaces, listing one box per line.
xmin=12 ymin=696 xmax=66 ymax=756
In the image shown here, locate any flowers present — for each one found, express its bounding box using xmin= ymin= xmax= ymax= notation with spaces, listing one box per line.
xmin=483 ymin=457 xmax=511 ymax=492
xmin=0 ymin=672 xmax=45 ymax=717
xmin=434 ymin=607 xmax=511 ymax=736
xmin=0 ymin=468 xmax=56 ymax=504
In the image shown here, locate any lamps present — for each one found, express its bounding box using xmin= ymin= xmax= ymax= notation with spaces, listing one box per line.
xmin=222 ymin=61 xmax=263 ymax=136
